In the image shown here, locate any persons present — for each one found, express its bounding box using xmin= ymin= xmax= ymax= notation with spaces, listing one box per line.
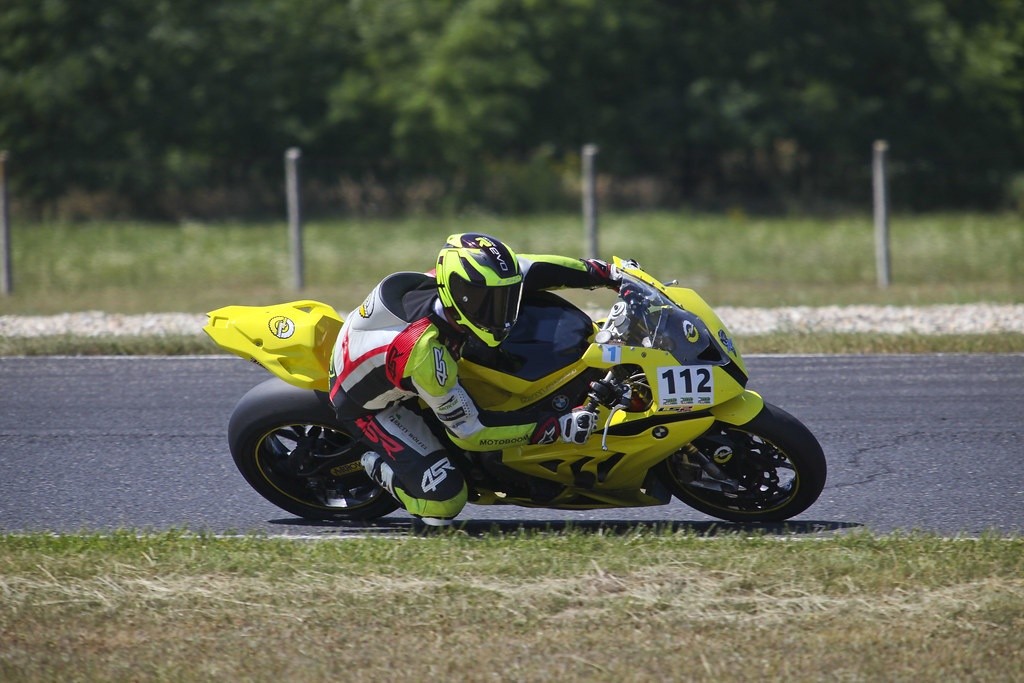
xmin=328 ymin=233 xmax=645 ymax=521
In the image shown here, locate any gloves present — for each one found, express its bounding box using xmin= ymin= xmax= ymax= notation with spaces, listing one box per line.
xmin=531 ymin=406 xmax=599 ymax=446
xmin=585 ymin=257 xmax=641 ymax=297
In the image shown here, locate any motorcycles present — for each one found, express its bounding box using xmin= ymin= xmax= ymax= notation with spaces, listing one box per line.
xmin=203 ymin=254 xmax=830 ymax=524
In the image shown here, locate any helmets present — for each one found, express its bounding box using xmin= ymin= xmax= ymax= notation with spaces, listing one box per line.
xmin=436 ymin=233 xmax=523 ymax=347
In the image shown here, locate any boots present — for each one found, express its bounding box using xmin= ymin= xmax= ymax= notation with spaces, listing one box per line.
xmin=361 ymin=451 xmax=403 ymax=506
